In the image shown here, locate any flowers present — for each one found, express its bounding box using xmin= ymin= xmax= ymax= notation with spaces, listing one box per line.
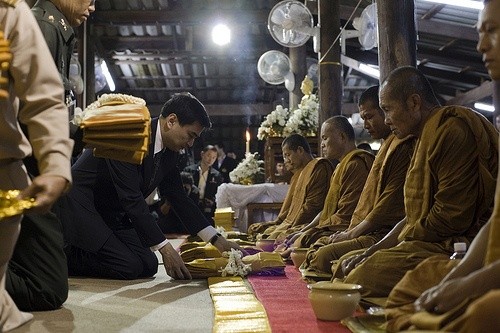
xmin=257 ymin=104 xmax=289 ymax=141
xmin=228 ymin=153 xmax=266 ymax=184
xmin=281 ymin=95 xmax=320 ymax=139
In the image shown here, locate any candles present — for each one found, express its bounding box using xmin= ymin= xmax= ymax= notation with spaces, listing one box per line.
xmin=245 ymin=130 xmax=251 ymax=153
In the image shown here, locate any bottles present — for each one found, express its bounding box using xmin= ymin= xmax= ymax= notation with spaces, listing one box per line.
xmin=449 ymin=242 xmax=467 ymax=262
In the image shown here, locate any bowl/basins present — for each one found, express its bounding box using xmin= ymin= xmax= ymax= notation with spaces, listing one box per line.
xmin=256 ymin=239 xmax=275 ymax=253
xmin=290 ymin=247 xmax=310 ymax=265
xmin=307 ymin=283 xmax=362 ymax=321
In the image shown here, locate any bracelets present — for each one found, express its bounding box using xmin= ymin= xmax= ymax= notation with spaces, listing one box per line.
xmin=211 ymin=233 xmax=222 ymax=245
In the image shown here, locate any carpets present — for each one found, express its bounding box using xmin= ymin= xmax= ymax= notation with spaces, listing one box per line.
xmin=246 ymin=263 xmax=363 ymax=332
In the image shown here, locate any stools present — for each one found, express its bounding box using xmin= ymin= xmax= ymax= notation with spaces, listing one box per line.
xmin=247 ymin=203 xmax=284 ymax=233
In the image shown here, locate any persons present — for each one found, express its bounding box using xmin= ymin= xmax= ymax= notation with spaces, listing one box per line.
xmin=4 ymin=0 xmax=95 ymax=312
xmin=52 ymin=90 xmax=247 ymax=280
xmin=249 ymin=67 xmax=500 ymax=304
xmin=0 ymin=0 xmax=74 ymax=333
xmin=382 ymin=0 xmax=500 ymax=333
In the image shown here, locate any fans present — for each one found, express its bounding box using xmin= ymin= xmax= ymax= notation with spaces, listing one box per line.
xmin=268 ymin=1 xmax=319 ymax=52
xmin=257 ymin=49 xmax=295 ymax=92
xmin=340 ymin=3 xmax=377 ymax=56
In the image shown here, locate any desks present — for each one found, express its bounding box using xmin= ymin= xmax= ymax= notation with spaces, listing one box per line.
xmin=263 ymin=138 xmax=321 ymax=185
xmin=216 ymin=182 xmax=290 ymax=232
